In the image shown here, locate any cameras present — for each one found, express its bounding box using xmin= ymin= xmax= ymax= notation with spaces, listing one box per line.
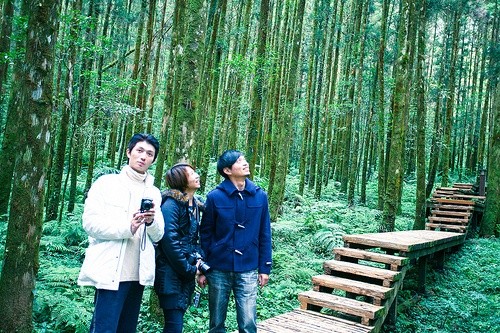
xmin=188 ymin=252 xmax=211 ymax=276
xmin=140 ymin=199 xmax=153 ymax=213
xmin=193 ymin=293 xmax=201 ymax=307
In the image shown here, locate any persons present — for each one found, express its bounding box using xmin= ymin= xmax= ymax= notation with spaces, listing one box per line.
xmin=197 ymin=150 xmax=274 ymax=333
xmin=77 ymin=132 xmax=166 ymax=333
xmin=153 ymin=163 xmax=201 ymax=333
